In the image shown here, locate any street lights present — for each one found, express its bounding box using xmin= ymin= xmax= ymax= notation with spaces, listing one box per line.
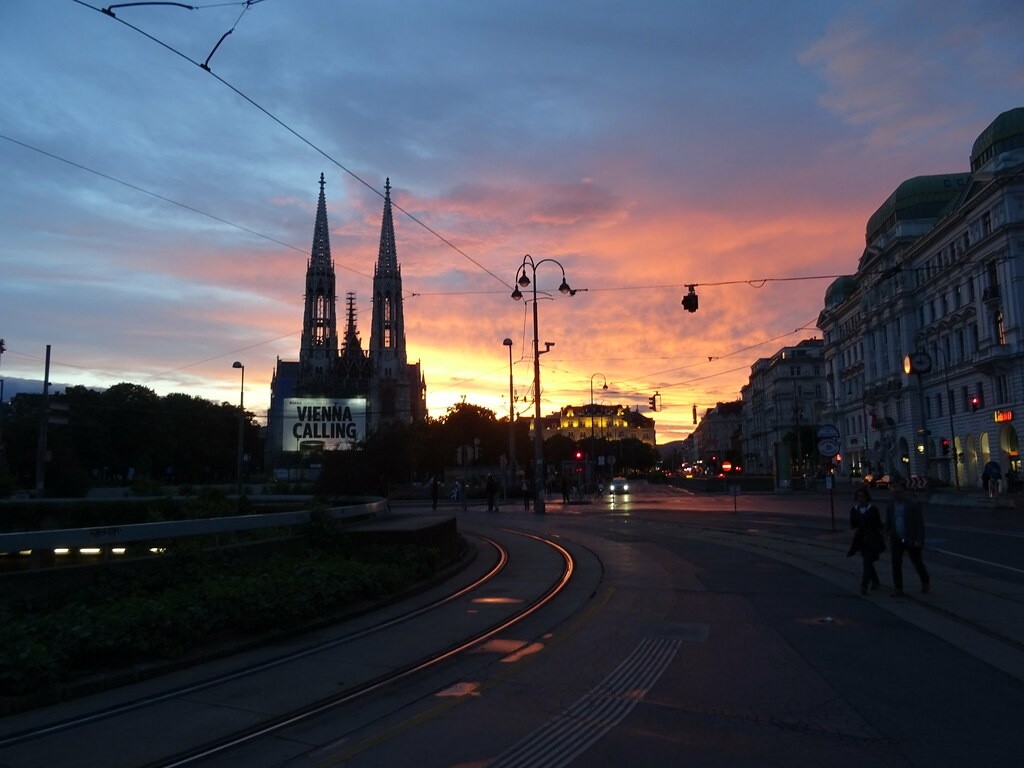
xmin=591 ymin=373 xmax=609 ymax=495
xmin=503 ymin=338 xmax=517 ymax=498
xmin=601 ymin=399 xmax=615 ymax=484
xmin=923 ymin=347 xmax=960 ymax=491
xmin=232 ymin=361 xmax=244 ymax=494
xmin=511 ymin=254 xmax=571 ymax=514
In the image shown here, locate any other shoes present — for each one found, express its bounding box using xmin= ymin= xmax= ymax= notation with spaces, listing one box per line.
xmin=889 ymin=589 xmax=904 ymax=596
xmin=922 ymin=581 xmax=931 ymax=593
xmin=860 ymin=585 xmax=866 ymax=593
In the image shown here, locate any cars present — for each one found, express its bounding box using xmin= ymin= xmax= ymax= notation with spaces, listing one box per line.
xmin=609 ymin=476 xmax=630 ymax=496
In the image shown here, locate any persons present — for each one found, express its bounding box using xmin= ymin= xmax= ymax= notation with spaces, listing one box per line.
xmin=561 ymin=472 xmax=605 ymax=504
xmin=431 ymin=477 xmax=441 ymax=512
xmin=1005 ymin=469 xmax=1024 ymax=493
xmin=486 ymin=473 xmax=500 ymax=512
xmin=885 ymin=487 xmax=930 ymax=598
xmin=460 ymin=481 xmax=468 ymax=511
xmin=521 ymin=471 xmax=557 ymax=510
xmin=846 ymin=487 xmax=886 ymax=599
xmin=888 ymin=468 xmax=897 ymax=491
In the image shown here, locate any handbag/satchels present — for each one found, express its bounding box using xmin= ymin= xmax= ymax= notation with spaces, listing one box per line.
xmin=876 ymin=532 xmax=887 ymax=553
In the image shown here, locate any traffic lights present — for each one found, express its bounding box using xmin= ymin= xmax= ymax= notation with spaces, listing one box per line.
xmin=575 ymin=449 xmax=584 ymax=461
xmin=649 ymin=396 xmax=656 ymax=412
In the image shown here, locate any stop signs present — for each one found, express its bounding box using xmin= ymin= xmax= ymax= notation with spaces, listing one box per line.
xmin=722 ymin=461 xmax=732 ymax=472
